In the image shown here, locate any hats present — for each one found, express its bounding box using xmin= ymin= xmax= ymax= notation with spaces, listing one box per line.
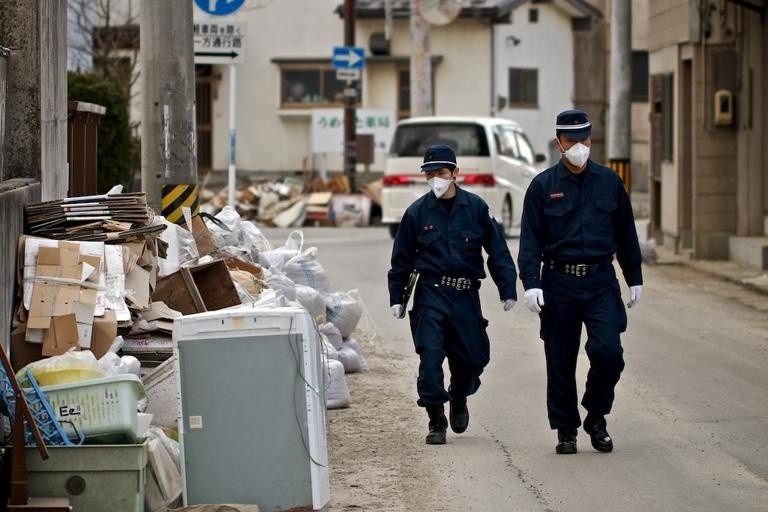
xmin=556 ymin=110 xmax=592 ymax=139
xmin=421 ymin=145 xmax=456 ymax=171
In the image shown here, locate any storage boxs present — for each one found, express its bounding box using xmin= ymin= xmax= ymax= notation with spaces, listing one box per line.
xmin=150 ymin=258 xmax=242 ymax=316
xmin=19 ymin=436 xmax=151 ymax=512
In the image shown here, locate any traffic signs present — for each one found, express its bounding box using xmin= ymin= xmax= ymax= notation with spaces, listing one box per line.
xmin=186 ymin=20 xmax=247 ymax=68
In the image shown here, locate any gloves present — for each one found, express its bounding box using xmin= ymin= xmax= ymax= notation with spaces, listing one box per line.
xmin=504 ymin=299 xmax=515 ymax=312
xmin=524 ymin=288 xmax=544 ymax=312
xmin=391 ymin=304 xmax=405 ymax=319
xmin=627 ymin=285 xmax=641 ymax=308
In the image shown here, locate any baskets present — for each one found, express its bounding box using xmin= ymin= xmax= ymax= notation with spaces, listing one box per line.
xmin=6 ymin=374 xmax=148 ymax=443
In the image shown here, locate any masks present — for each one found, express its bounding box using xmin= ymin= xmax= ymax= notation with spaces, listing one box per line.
xmin=564 ymin=142 xmax=590 ymax=169
xmin=427 ymin=176 xmax=452 ymax=198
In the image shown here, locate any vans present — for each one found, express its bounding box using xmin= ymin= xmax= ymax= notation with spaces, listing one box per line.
xmin=379 ymin=114 xmax=546 ymax=243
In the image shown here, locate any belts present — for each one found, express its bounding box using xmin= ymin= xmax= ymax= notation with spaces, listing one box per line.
xmin=545 ymin=259 xmax=600 ymax=277
xmin=431 ymin=274 xmax=476 ymax=292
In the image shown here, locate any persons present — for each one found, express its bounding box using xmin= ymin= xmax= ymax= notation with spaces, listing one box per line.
xmin=387 ymin=145 xmax=517 ymax=444
xmin=516 ymin=110 xmax=643 ymax=454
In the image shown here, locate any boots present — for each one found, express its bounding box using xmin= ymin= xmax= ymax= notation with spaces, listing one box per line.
xmin=426 ymin=403 xmax=448 ymax=444
xmin=448 ymin=385 xmax=469 ymax=433
xmin=556 ymin=428 xmax=577 ymax=454
xmin=583 ymin=411 xmax=613 ymax=451
